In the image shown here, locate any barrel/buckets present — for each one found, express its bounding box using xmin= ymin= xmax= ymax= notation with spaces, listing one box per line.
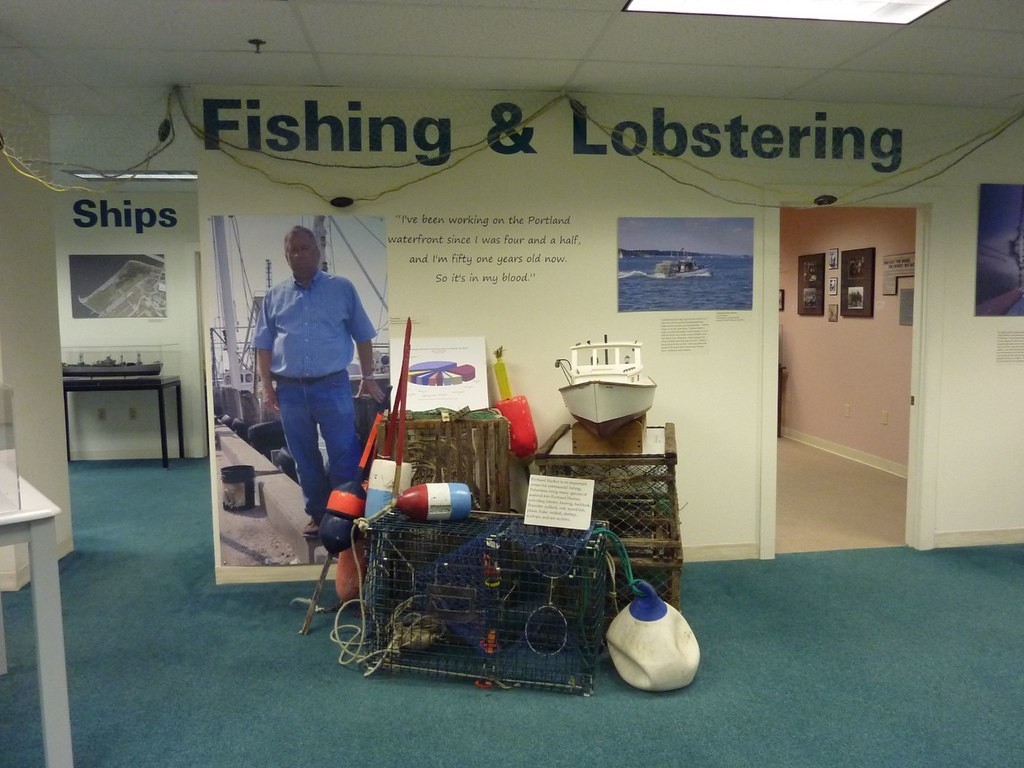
xmin=220 ymin=465 xmax=255 ymax=513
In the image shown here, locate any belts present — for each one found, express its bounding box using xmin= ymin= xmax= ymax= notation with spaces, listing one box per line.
xmin=278 ymin=370 xmax=345 ymax=385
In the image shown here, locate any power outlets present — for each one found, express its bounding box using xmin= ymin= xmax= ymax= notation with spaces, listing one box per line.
xmin=128 ymin=407 xmax=137 ymax=420
xmin=845 ymin=403 xmax=850 ymax=417
xmin=880 ymin=411 xmax=888 ymax=425
xmin=97 ymin=407 xmax=106 ymax=421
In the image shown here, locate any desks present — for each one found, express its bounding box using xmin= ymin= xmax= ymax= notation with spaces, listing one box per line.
xmin=0 ymin=475 xmax=74 ymax=768
xmin=63 ymin=375 xmax=185 ymax=471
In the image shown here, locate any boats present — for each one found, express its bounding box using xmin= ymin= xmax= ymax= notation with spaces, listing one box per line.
xmin=207 ymin=216 xmax=392 ymax=458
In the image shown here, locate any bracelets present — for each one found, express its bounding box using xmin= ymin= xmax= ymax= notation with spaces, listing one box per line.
xmin=361 ymin=375 xmax=374 ymax=380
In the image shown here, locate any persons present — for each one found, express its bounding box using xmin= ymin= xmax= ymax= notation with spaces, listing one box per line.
xmin=803 ymin=293 xmax=816 ymax=306
xmin=805 ymin=266 xmax=816 ymax=281
xmin=250 ymin=225 xmax=385 ymax=536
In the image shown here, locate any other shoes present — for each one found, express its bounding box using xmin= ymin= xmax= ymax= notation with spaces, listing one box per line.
xmin=302 ymin=515 xmax=320 ymax=540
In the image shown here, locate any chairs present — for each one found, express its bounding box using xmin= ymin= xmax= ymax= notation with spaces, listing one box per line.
xmin=571 ymin=420 xmax=656 ymax=539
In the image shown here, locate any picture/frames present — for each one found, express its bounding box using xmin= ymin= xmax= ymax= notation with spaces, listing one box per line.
xmin=779 ymin=289 xmax=784 ymax=310
